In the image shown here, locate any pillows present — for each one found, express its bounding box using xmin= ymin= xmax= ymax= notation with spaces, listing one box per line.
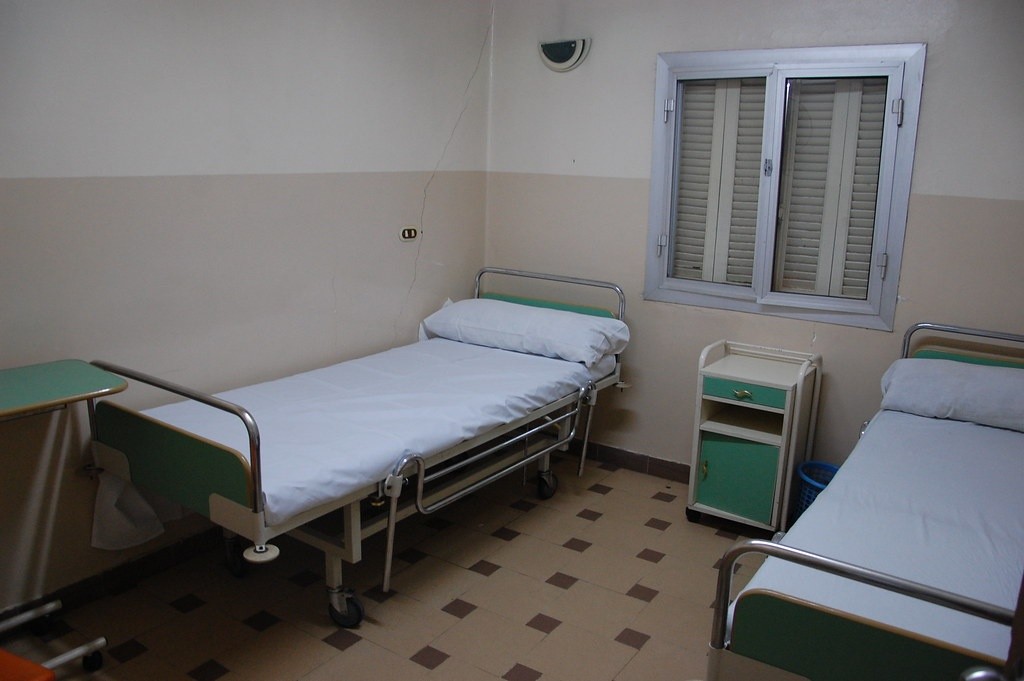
xmin=880 ymin=358 xmax=1024 ymax=433
xmin=417 ymin=297 xmax=630 ymax=367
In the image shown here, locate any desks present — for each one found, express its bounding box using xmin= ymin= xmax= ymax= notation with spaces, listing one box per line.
xmin=0 ymin=359 xmax=131 ymax=681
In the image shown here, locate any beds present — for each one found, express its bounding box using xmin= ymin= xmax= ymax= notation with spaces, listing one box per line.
xmin=87 ymin=267 xmax=626 ymax=628
xmin=709 ymin=320 xmax=1024 ymax=681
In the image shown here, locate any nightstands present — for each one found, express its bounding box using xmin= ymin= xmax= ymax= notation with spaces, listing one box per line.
xmin=687 ymin=339 xmax=824 ymax=532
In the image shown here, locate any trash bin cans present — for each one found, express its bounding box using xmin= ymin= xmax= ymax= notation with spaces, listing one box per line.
xmin=796 ymin=459 xmax=841 ymax=525
